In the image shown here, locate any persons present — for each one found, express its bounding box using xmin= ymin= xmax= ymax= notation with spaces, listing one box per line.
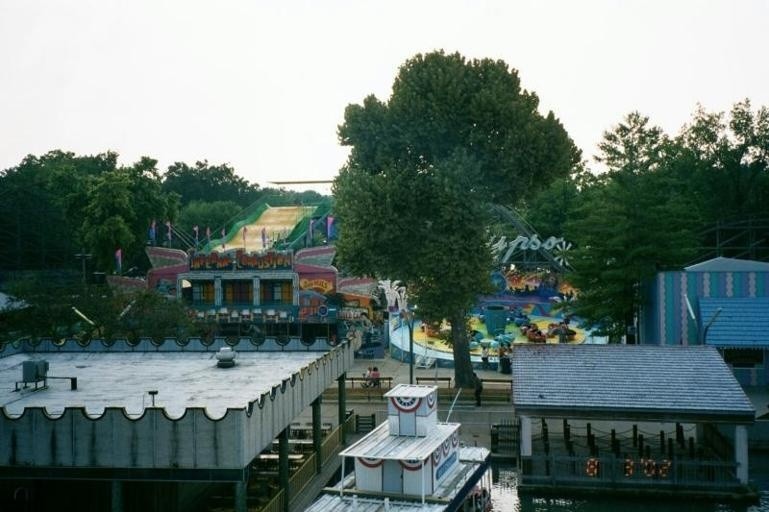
xmin=499 ymin=342 xmax=507 ymax=356
xmin=481 ymin=346 xmax=489 ymax=369
xmin=372 ymin=367 xmax=381 ymax=386
xmin=364 ymin=366 xmax=374 ymax=387
